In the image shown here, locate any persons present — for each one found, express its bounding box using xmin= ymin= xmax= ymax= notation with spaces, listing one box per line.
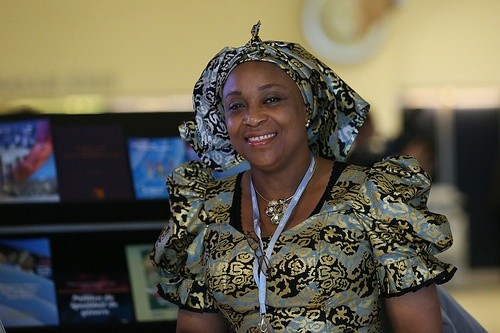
xmin=148 ymin=21 xmax=456 ymax=333
xmin=347 ymin=107 xmax=470 ymax=266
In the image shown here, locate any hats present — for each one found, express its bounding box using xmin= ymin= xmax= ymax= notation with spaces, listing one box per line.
xmin=179 ymin=21 xmax=371 ymax=173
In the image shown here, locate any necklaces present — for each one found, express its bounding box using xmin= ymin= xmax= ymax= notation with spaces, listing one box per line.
xmin=254 ymin=186 xmax=293 ymax=225
